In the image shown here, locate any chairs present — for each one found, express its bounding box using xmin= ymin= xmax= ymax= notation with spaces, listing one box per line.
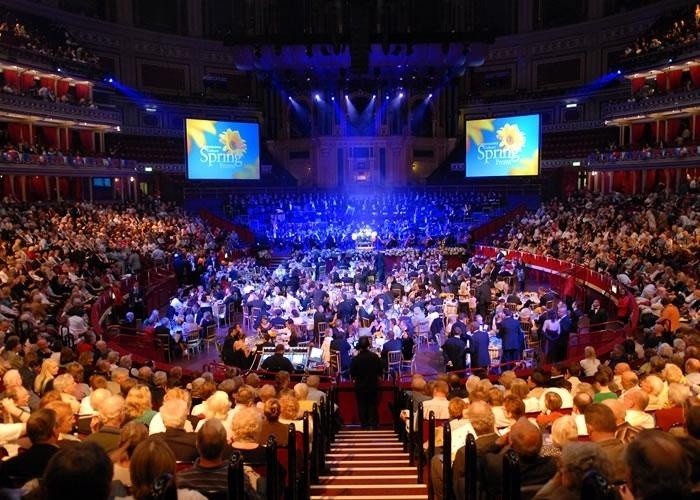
xmin=0 ymin=271 xmax=700 ymax=500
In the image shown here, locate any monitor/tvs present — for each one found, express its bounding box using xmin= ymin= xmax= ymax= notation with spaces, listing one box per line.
xmin=255 ymin=355 xmax=309 ymax=368
